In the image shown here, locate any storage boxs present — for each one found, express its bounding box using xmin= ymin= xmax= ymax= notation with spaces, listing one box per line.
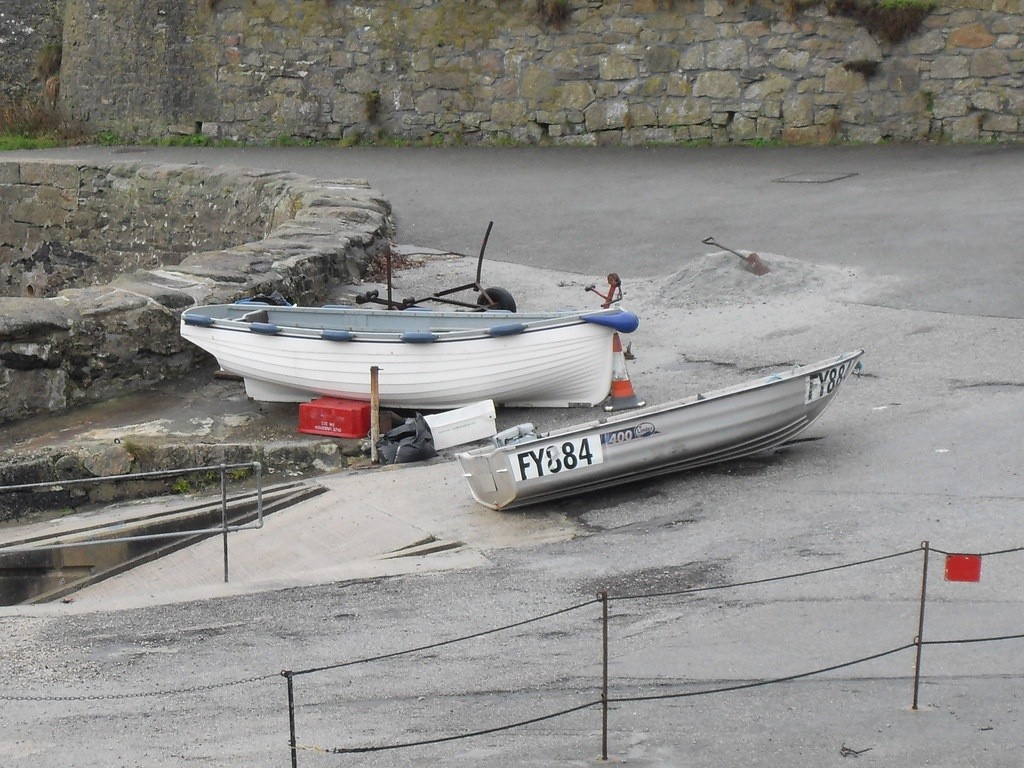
xmin=298 ymin=396 xmax=371 ymax=438
xmin=425 ymin=398 xmax=498 ymax=450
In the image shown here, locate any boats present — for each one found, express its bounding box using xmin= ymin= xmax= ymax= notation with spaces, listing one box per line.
xmin=180 ymin=302 xmax=640 ymax=411
xmin=455 ymin=347 xmax=867 ymax=511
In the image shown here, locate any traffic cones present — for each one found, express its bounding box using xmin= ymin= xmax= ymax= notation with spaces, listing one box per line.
xmin=602 ymin=332 xmax=648 ymax=412
